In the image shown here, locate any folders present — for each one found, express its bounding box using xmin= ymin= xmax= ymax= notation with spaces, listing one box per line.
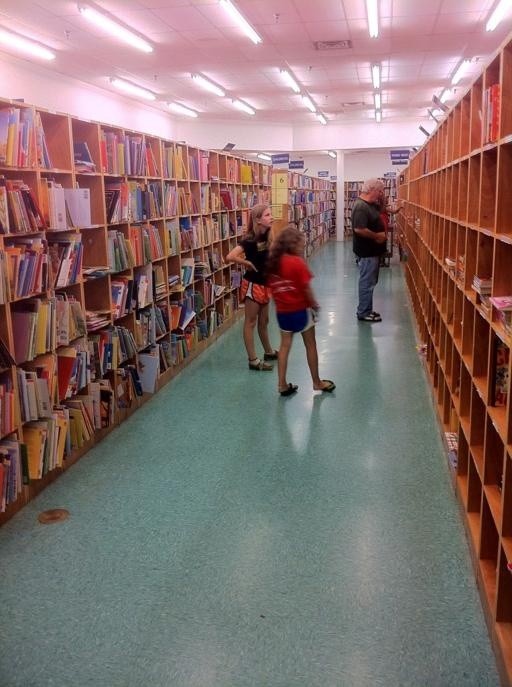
xmin=116 ymin=276 xmax=134 ymax=318
xmin=130 ymin=181 xmax=162 ymax=220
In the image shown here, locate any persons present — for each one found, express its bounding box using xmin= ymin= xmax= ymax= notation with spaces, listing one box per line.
xmin=288 ymin=172 xmax=336 ymax=261
xmin=162 ymin=138 xmax=271 ymax=372
xmin=271 ymin=228 xmax=335 ymax=396
xmin=0 ymin=105 xmax=167 ymax=512
xmin=351 ymin=176 xmax=390 ymax=323
xmin=226 ymin=204 xmax=281 ymax=373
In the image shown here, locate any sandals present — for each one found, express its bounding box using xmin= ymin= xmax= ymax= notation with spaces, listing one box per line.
xmin=248 ymin=357 xmax=273 ymax=372
xmin=263 ymin=350 xmax=279 ymax=361
xmin=356 ymin=311 xmax=382 ymax=322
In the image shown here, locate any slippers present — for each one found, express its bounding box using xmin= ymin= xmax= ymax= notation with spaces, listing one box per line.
xmin=280 ymin=383 xmax=299 ymax=397
xmin=322 ymin=379 xmax=336 ymax=392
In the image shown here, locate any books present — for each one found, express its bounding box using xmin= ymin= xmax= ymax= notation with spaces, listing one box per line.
xmin=444 ymin=251 xmax=512 ymax=340
xmin=481 ymin=83 xmax=500 ymax=145
xmin=413 ymin=340 xmax=427 ymax=365
xmin=443 ymin=429 xmax=463 ymax=469
xmin=343 ymin=178 xmax=420 ymax=269
xmin=489 ymin=344 xmax=511 ymax=410
